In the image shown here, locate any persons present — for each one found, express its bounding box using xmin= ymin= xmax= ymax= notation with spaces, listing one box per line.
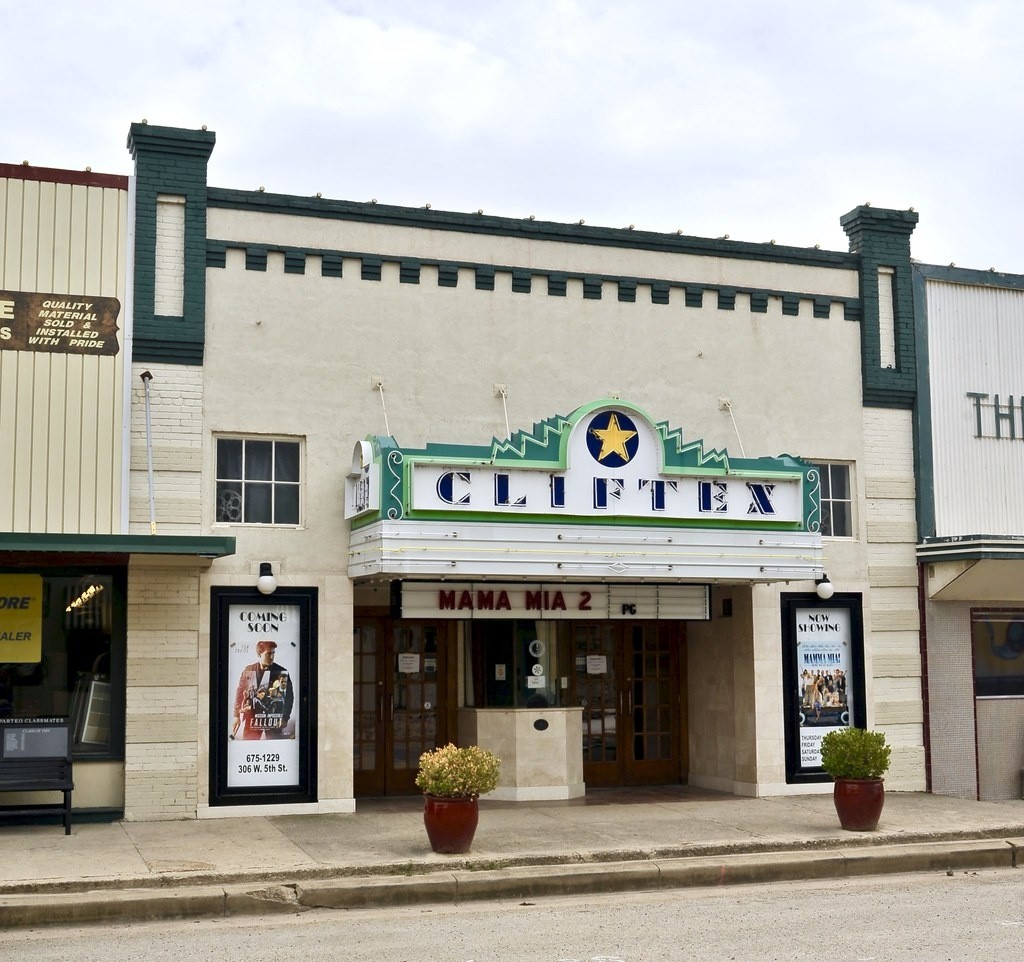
xmin=800 ymin=669 xmax=847 ymax=721
xmin=232 ymin=641 xmax=294 ymax=740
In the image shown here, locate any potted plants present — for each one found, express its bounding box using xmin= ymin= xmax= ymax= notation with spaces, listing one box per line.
xmin=820 ymin=728 xmax=892 ymax=832
xmin=415 ymin=742 xmax=501 ymax=854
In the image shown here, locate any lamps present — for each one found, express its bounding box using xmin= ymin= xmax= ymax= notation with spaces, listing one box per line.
xmin=258 ymin=563 xmax=277 ymax=594
xmin=817 ymin=574 xmax=833 ymax=598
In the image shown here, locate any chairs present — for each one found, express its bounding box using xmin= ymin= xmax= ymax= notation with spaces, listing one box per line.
xmin=0 ymin=717 xmax=75 ymax=836
